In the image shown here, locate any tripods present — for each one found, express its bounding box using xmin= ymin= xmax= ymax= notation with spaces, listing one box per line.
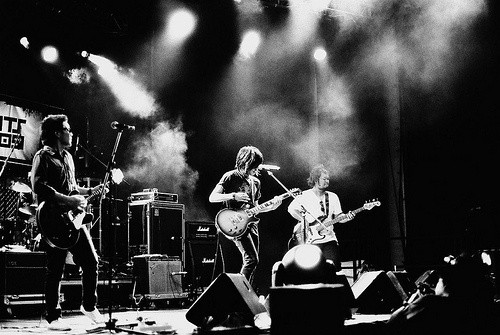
xmin=85 ymin=132 xmax=175 ymax=335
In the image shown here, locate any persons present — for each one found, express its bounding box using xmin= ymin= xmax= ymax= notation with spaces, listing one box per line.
xmin=31 ymin=114 xmax=109 ymax=330
xmin=288 ymin=165 xmax=355 ymax=265
xmin=20 ymin=171 xmax=37 ymax=207
xmin=209 ymin=146 xmax=282 ymax=286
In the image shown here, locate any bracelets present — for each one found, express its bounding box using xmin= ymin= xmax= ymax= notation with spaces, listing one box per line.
xmin=233 ymin=193 xmax=235 ymax=200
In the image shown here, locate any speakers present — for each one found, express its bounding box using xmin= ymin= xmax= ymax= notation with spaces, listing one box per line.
xmin=351 ymin=270 xmax=403 ymax=313
xmin=186 ymin=240 xmax=216 ymax=287
xmin=132 ymin=255 xmax=183 ymax=299
xmin=186 ymin=273 xmax=266 ymax=329
xmin=0 ymin=252 xmax=48 ymax=318
xmin=268 ymin=284 xmax=348 ymax=335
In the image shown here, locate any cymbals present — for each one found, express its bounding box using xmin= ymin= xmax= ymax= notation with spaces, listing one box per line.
xmin=7 ymin=180 xmax=32 ymax=194
xmin=17 ymin=204 xmax=38 ymax=216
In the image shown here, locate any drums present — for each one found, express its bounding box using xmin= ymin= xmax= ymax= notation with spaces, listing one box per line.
xmin=27 ymin=217 xmax=39 ymax=245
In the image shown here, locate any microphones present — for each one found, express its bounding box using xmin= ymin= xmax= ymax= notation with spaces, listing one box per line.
xmin=110 ymin=121 xmax=135 ymax=131
xmin=258 ymin=164 xmax=281 ymax=171
xmin=171 ymin=272 xmax=187 ymax=276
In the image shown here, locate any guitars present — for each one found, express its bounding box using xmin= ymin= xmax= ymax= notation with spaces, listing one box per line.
xmin=213 ymin=187 xmax=303 ymax=241
xmin=37 ymin=184 xmax=112 ymax=251
xmin=287 ymin=197 xmax=381 ymax=253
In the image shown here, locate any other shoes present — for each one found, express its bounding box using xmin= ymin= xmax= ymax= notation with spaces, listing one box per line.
xmin=80 ymin=303 xmax=106 ymax=324
xmin=48 ymin=317 xmax=73 ymax=331
